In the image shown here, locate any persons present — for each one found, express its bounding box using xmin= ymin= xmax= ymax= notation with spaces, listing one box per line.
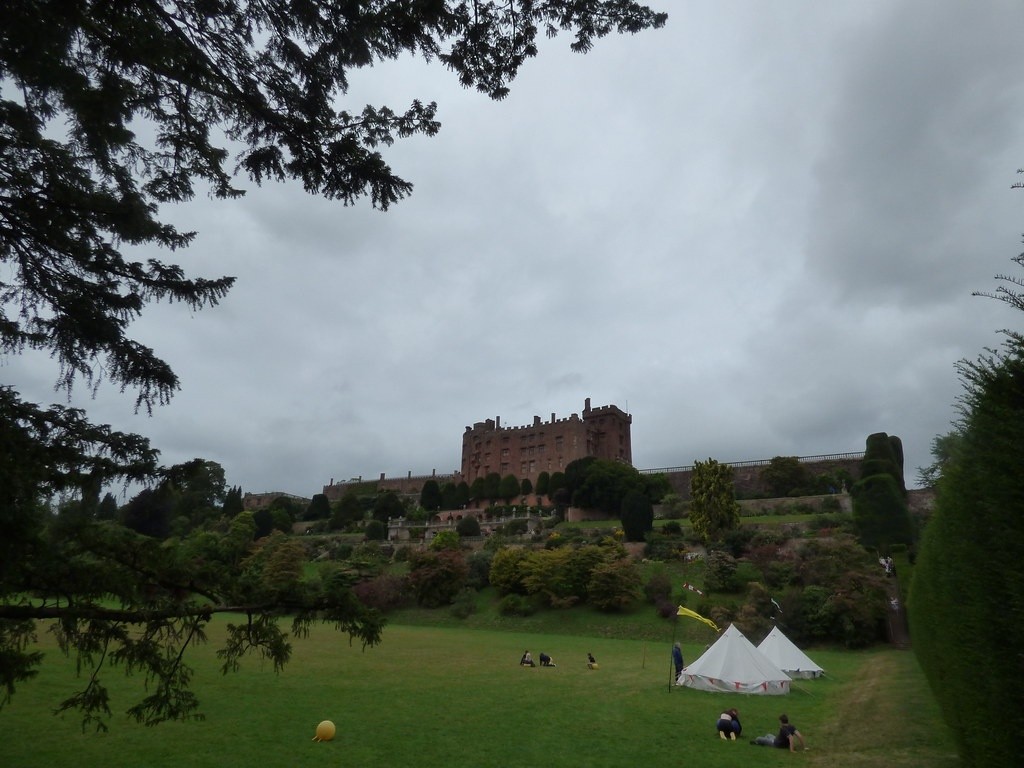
xmin=749 ymin=714 xmax=809 ymax=753
xmin=671 ymin=643 xmax=685 ymax=681
xmin=520 ymin=650 xmax=535 ymax=667
xmin=879 ymin=556 xmax=894 ymax=577
xmin=717 ymin=707 xmax=742 ymax=740
xmin=539 ymin=652 xmax=557 ymax=667
xmin=586 ymin=653 xmax=595 ymax=670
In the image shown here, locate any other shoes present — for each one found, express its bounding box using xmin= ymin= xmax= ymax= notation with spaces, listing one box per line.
xmin=720 ymin=731 xmax=726 ymax=739
xmin=730 ymin=732 xmax=736 ymax=741
xmin=750 ymin=741 xmax=757 ymax=745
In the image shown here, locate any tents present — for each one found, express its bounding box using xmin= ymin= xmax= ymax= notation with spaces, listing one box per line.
xmin=678 ymin=622 xmax=792 ymax=697
xmin=754 ymin=626 xmax=824 ymax=679
